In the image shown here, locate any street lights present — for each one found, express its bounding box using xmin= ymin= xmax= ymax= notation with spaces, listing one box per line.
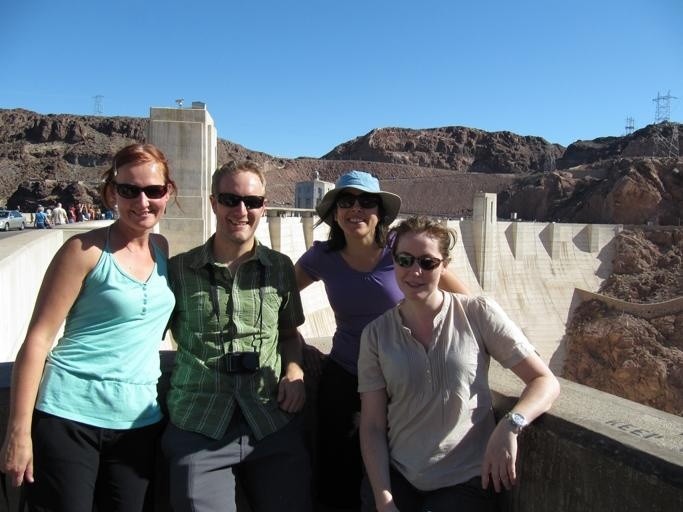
xmin=175 ymin=98 xmax=184 ymax=108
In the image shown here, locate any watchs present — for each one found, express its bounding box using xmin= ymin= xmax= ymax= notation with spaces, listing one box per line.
xmin=503 ymin=412 xmax=528 ymax=438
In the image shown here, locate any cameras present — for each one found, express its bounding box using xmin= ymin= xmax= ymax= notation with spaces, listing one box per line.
xmin=226 ymin=351 xmax=260 ymax=373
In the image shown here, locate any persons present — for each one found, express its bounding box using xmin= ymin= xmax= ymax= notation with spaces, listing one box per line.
xmin=17 ymin=202 xmax=115 ymax=229
xmin=291 ymin=169 xmax=472 ymax=512
xmin=357 ymin=216 xmax=560 ymax=511
xmin=167 ymin=162 xmax=317 ymax=512
xmin=0 ymin=143 xmax=177 ymax=512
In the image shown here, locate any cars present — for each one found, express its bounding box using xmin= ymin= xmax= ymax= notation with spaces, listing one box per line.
xmin=0 ymin=209 xmax=27 ymax=232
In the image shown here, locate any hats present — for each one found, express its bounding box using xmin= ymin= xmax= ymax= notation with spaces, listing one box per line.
xmin=317 ymin=171 xmax=403 ymax=227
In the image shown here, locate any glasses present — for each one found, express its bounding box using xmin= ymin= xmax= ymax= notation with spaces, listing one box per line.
xmin=214 ymin=192 xmax=266 ymax=209
xmin=108 ymin=178 xmax=169 ymax=198
xmin=337 ymin=191 xmax=380 ymax=208
xmin=393 ymin=252 xmax=445 ymax=269
